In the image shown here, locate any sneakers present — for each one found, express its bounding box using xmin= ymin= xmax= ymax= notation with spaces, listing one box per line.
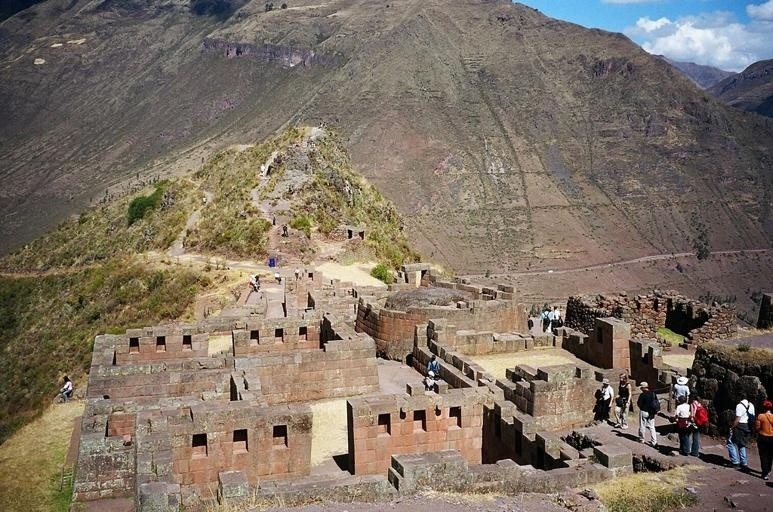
xmin=638 ymin=436 xmax=657 ymax=448
xmin=615 ymin=423 xmax=627 ymax=429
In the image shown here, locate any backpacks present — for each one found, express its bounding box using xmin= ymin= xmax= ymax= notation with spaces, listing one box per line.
xmin=741 ymin=401 xmax=755 ymax=427
xmin=693 ymin=402 xmax=708 ymax=426
xmin=543 ymin=312 xmax=550 ymax=325
xmin=431 ymin=362 xmax=440 ymax=380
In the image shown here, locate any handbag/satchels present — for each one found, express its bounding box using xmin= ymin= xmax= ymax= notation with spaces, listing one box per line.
xmin=615 ymin=397 xmax=623 ymax=407
xmin=650 ymin=400 xmax=660 ymax=413
xmin=679 ymin=417 xmax=689 ymax=427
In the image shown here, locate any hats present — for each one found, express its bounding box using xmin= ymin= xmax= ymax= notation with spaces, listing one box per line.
xmin=639 ymin=382 xmax=648 ymax=388
xmin=603 ymin=379 xmax=610 ymax=385
xmin=763 ymin=400 xmax=772 ymax=409
xmin=428 ymin=371 xmax=434 ymax=377
xmin=677 ymin=377 xmax=688 ymax=384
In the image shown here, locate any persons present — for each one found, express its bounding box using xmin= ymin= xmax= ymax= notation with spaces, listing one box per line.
xmin=637 ymin=381 xmax=657 ymax=448
xmin=250 ymin=275 xmax=260 ymax=292
xmin=756 ymin=400 xmax=773 ymax=480
xmin=672 ymin=377 xmax=702 ymax=457
xmin=202 ymin=196 xmax=207 ymax=205
xmin=615 ymin=373 xmax=632 ymax=429
xmin=294 ymin=266 xmax=299 ymax=281
xmin=275 ymin=272 xmax=281 ymax=284
xmin=60 ymin=376 xmax=72 ymax=403
xmin=727 ymin=392 xmax=756 ymax=468
xmin=528 ymin=302 xmax=564 ymax=333
xmin=593 ymin=379 xmax=614 ymax=423
xmin=283 ymin=224 xmax=288 ymax=237
xmin=273 ymin=217 xmax=275 ymax=224
xmin=424 ymin=355 xmax=441 ymax=391
